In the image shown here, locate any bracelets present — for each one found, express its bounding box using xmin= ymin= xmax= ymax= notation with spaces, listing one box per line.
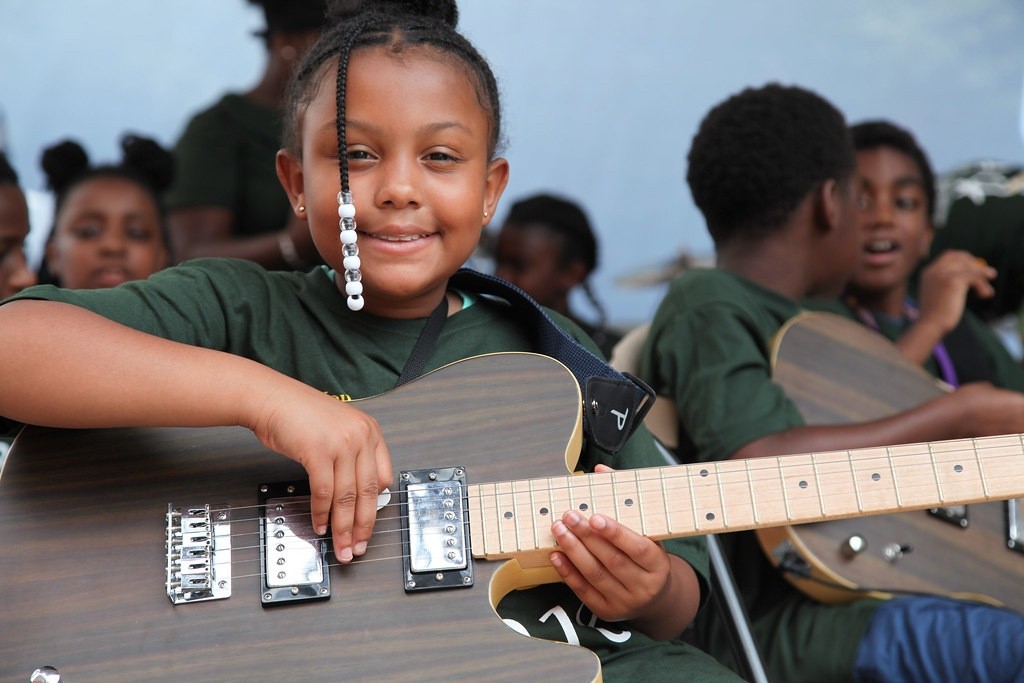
xmin=277 ymin=233 xmax=304 ymax=268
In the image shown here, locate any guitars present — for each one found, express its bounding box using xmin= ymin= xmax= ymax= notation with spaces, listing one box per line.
xmin=757 ymin=309 xmax=1024 ymax=611
xmin=0 ymin=349 xmax=1022 ymax=683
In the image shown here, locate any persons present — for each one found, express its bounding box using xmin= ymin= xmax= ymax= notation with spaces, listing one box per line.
xmin=0 ymin=151 xmax=36 ymax=301
xmin=495 ymin=197 xmax=624 ymax=362
xmin=164 ymin=0 xmax=329 ymax=271
xmin=0 ymin=1 xmax=747 ymax=683
xmin=812 ymin=121 xmax=1024 ymax=398
xmin=42 ymin=135 xmax=175 ymax=289
xmin=636 ymin=84 xmax=1024 ymax=683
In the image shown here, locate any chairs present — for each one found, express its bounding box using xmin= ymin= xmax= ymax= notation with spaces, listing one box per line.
xmin=610 ymin=324 xmax=768 ymax=683
